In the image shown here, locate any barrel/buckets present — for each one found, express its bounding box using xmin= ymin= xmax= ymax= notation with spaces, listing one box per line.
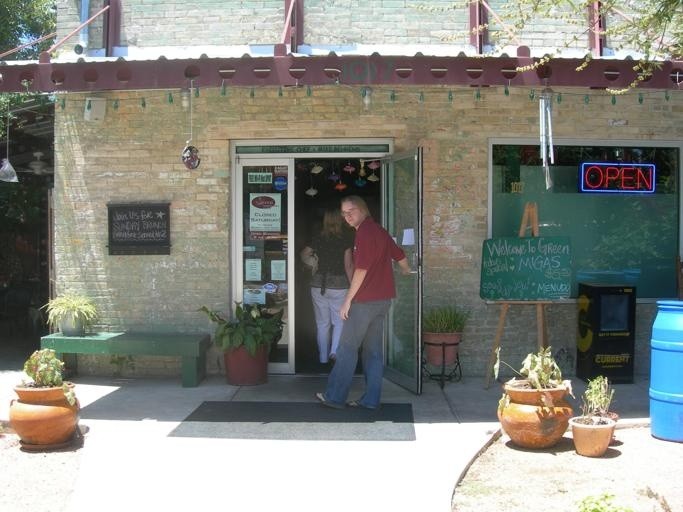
xmin=648 ymin=301 xmax=683 ymax=442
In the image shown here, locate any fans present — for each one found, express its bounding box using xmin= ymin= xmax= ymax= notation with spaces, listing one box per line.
xmin=13 ymin=151 xmax=57 ymax=177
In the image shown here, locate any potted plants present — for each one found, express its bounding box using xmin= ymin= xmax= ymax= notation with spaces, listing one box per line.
xmin=420 ymin=307 xmax=472 ymax=365
xmin=34 ymin=287 xmax=102 ymax=337
xmin=569 ymin=374 xmax=621 ymax=458
xmin=8 ymin=344 xmax=79 ymax=443
xmin=488 ymin=345 xmax=575 ymax=452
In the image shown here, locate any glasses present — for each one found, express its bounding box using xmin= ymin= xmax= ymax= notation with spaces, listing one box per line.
xmin=341 ymin=208 xmax=359 ymax=216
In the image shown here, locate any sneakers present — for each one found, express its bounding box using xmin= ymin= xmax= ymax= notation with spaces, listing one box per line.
xmin=328 ymin=352 xmax=337 ymax=369
xmin=313 ymin=392 xmax=346 ymax=409
xmin=346 ymin=399 xmax=365 ymax=408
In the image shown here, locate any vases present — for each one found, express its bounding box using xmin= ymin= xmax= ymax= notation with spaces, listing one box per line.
xmin=224 ymin=344 xmax=270 ymax=386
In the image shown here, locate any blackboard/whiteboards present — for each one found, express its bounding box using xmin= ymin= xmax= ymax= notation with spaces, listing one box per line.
xmin=106 ymin=203 xmax=172 ymax=255
xmin=479 ymin=236 xmax=572 ymax=301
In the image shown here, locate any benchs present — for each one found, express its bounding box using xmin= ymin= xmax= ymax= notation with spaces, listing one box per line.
xmin=39 ymin=330 xmax=211 ymax=389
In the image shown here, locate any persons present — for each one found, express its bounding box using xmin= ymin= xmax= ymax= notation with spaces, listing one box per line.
xmin=315 ymin=195 xmax=412 ymax=409
xmin=298 ymin=209 xmax=354 ymax=369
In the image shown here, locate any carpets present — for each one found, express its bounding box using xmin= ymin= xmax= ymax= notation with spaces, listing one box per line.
xmin=163 ymin=398 xmax=415 ymax=442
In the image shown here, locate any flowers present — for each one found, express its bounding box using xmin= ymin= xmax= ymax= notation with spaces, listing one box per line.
xmin=194 ymin=301 xmax=284 ymax=359
